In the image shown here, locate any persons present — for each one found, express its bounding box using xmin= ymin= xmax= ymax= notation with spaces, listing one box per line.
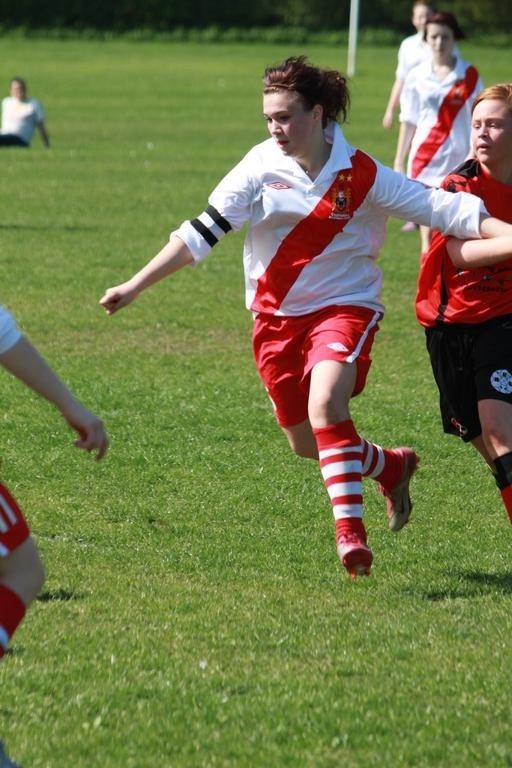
xmin=0 ymin=76 xmax=57 ymax=147
xmin=392 ymin=8 xmax=485 ymax=262
xmin=378 ymin=0 xmax=463 ymax=131
xmin=1 ymin=295 xmax=112 ymax=651
xmin=413 ymin=82 xmax=512 ymax=526
xmin=96 ymin=52 xmax=511 ymax=578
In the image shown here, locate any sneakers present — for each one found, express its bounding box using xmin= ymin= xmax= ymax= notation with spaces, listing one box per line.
xmin=380 ymin=447 xmax=420 ymax=533
xmin=336 ymin=532 xmax=374 ymax=580
